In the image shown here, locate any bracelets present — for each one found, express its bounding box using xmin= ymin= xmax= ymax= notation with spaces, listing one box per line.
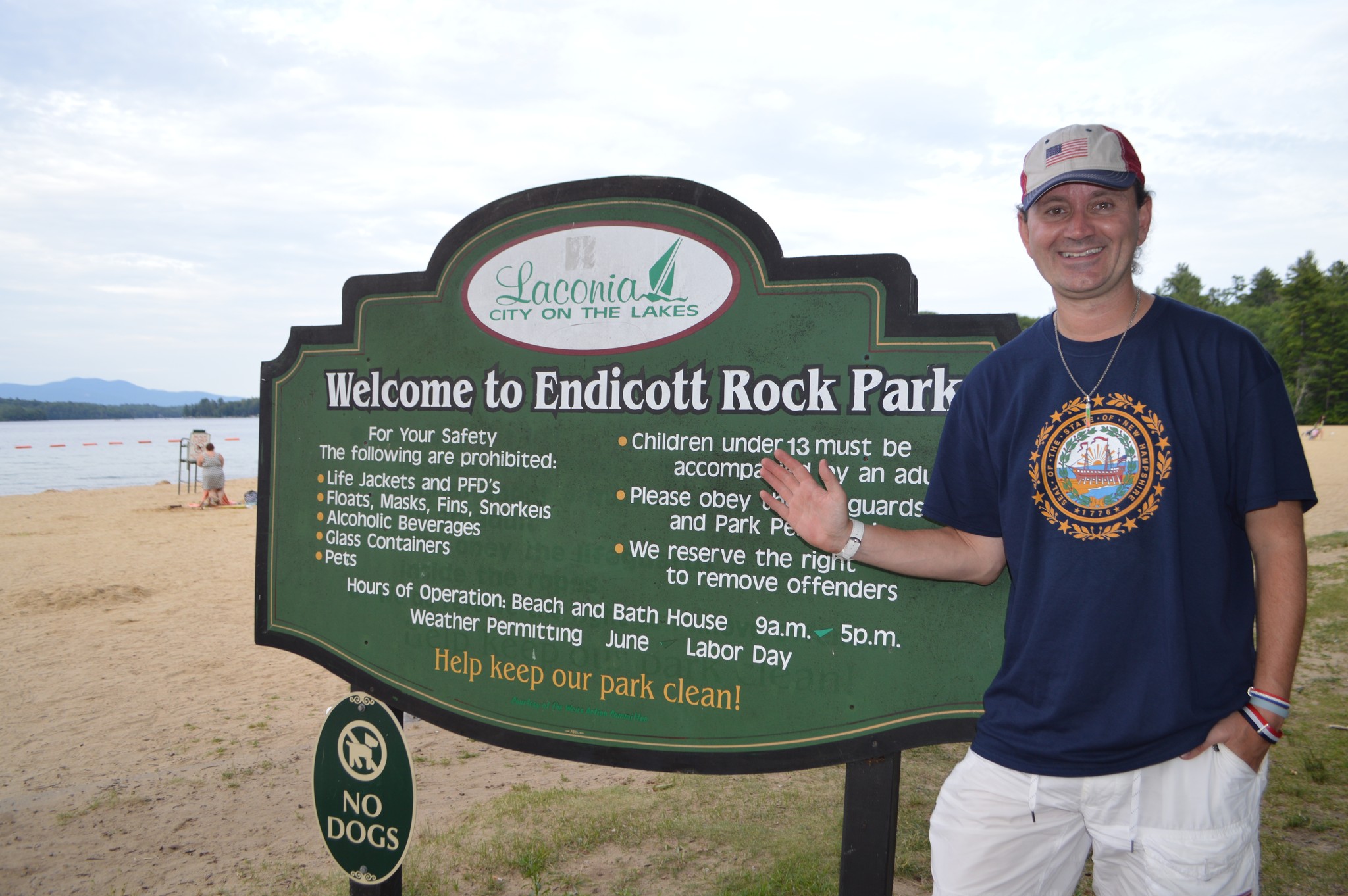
xmin=832 ymin=518 xmax=864 ymax=560
xmin=1237 ymin=703 xmax=1282 ymax=745
xmin=1247 ymin=687 xmax=1290 ymax=709
xmin=1249 ymin=696 xmax=1289 ymax=719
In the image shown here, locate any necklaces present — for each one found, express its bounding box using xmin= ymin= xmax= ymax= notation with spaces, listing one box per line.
xmin=1055 ymin=287 xmax=1140 ymax=428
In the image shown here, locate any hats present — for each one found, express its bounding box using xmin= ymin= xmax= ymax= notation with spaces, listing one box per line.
xmin=1022 ymin=125 xmax=1146 ymax=212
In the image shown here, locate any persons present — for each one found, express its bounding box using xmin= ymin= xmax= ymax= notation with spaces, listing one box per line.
xmin=197 ymin=443 xmax=224 ymax=506
xmin=759 ymin=125 xmax=1318 ymax=896
